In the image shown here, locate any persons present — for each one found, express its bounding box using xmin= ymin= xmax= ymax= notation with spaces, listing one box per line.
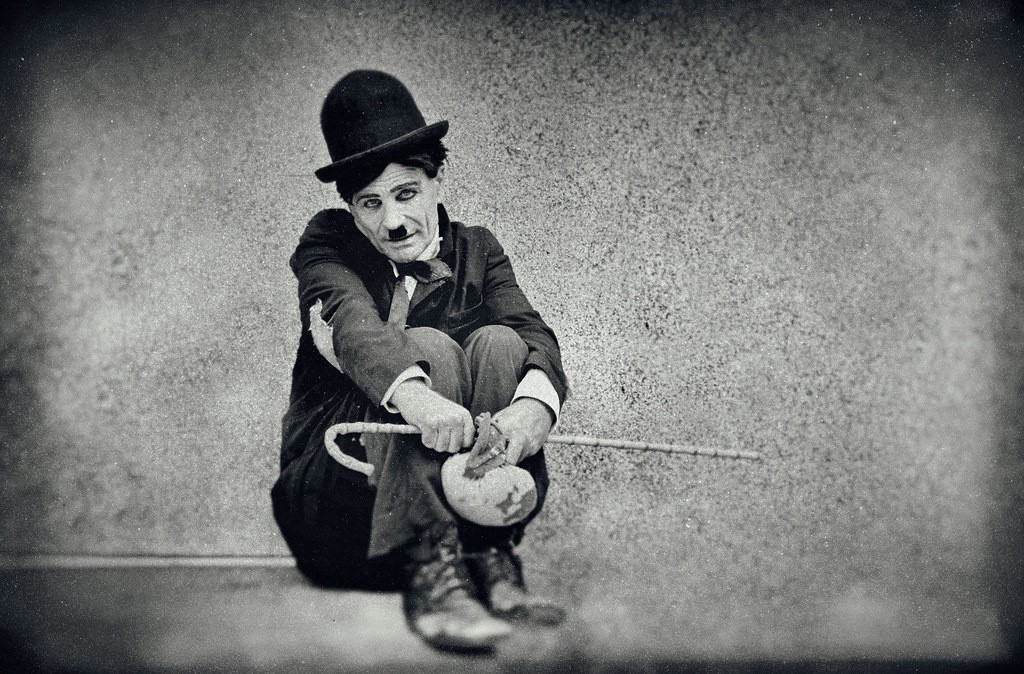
xmin=270 ymin=68 xmax=568 ymax=647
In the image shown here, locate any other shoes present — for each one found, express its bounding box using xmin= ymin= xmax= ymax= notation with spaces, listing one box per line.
xmin=404 ymin=555 xmax=513 ymax=654
xmin=465 ymin=547 xmax=566 ymax=630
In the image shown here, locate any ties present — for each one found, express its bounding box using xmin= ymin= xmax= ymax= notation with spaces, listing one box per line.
xmin=362 ymin=257 xmax=457 ymax=487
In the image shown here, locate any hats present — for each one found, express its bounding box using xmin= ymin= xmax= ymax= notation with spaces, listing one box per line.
xmin=315 ymin=69 xmax=450 ymax=186
